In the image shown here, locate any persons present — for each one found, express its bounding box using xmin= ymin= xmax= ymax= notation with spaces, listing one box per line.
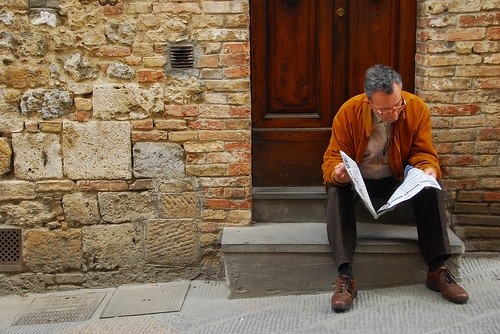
xmin=321 ymin=65 xmax=468 ymax=310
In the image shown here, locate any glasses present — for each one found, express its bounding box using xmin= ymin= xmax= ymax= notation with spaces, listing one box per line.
xmin=370 ymin=97 xmax=406 ymax=116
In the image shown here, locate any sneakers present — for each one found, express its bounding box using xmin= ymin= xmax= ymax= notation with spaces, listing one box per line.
xmin=426 ymin=266 xmax=469 ymax=303
xmin=331 ymin=275 xmax=358 ymax=312
